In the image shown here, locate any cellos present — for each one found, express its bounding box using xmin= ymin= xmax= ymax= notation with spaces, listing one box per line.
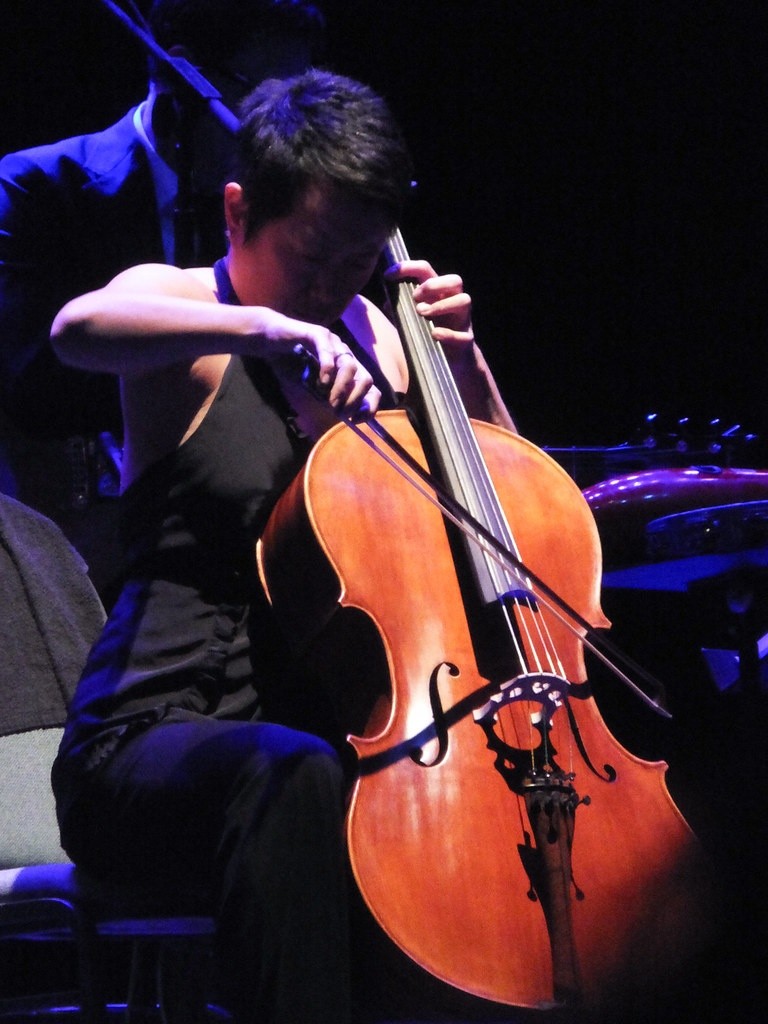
xmin=256 ymin=226 xmax=698 ymax=1024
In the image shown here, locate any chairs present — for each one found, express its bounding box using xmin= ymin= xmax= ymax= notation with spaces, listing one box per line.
xmin=0 ymin=496 xmax=237 ymax=1024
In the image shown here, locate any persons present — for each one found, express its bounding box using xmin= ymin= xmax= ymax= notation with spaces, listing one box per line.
xmin=49 ymin=67 xmax=520 ymax=1024
xmin=0 ymin=0 xmax=325 ymax=619
